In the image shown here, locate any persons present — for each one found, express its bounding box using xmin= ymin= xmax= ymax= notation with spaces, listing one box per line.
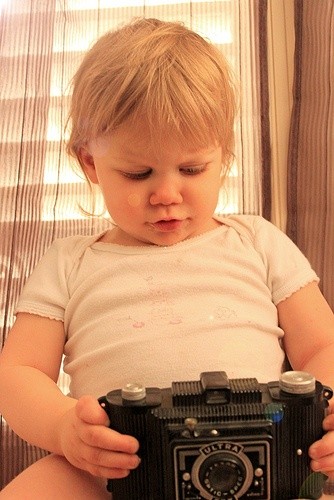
xmin=1 ymin=17 xmax=334 ymax=499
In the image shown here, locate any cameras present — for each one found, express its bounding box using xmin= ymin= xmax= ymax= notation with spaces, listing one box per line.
xmin=99 ymin=370 xmax=331 ymax=499
